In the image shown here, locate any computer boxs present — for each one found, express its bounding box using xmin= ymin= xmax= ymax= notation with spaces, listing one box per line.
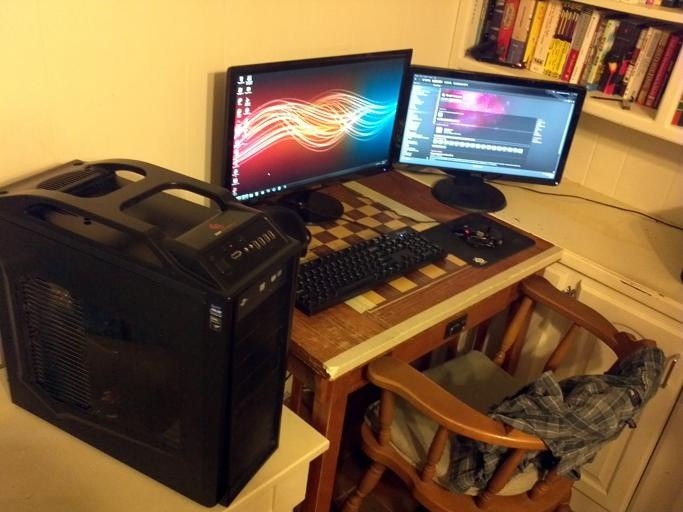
xmin=0 ymin=157 xmax=301 ymax=507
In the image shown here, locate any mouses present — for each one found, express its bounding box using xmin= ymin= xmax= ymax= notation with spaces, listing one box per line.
xmin=453 ymin=219 xmax=507 ymax=249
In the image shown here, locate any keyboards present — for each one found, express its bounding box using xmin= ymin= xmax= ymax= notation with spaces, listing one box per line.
xmin=291 ymin=224 xmax=449 ymax=316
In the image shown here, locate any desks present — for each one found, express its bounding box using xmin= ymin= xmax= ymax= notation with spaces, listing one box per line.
xmin=0 ymin=343 xmax=331 ymax=512
xmin=244 ymin=169 xmax=564 ymax=512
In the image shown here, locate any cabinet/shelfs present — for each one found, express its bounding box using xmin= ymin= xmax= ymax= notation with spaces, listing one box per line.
xmin=453 ymin=0 xmax=683 ymax=146
xmin=395 ymin=162 xmax=683 ymax=512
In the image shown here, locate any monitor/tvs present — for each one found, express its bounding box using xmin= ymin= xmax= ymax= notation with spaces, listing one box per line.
xmin=398 ymin=64 xmax=587 ymax=215
xmin=222 ymin=45 xmax=413 ymax=221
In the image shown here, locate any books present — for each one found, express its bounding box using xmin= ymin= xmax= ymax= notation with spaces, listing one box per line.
xmin=471 ymin=0 xmax=682 ymax=129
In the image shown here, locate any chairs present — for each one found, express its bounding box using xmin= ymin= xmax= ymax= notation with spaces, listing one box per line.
xmin=340 ymin=273 xmax=659 ymax=512
